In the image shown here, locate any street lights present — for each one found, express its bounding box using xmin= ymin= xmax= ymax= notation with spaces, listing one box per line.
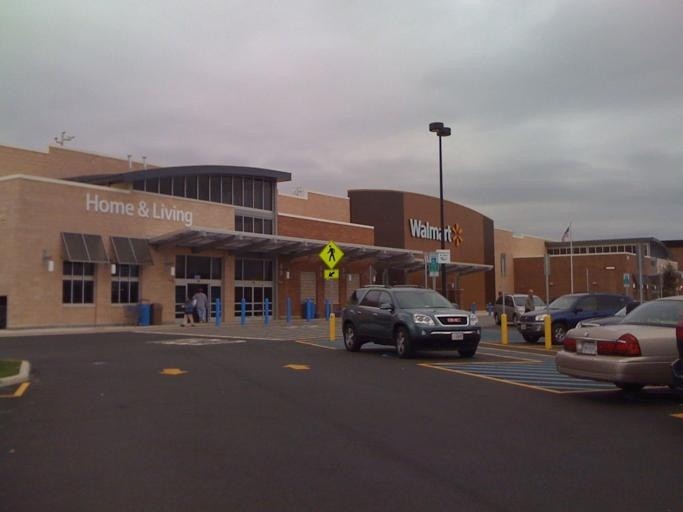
xmin=430 ymin=122 xmax=451 ymax=298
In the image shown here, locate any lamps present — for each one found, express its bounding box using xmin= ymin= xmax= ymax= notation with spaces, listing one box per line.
xmin=110 ymin=258 xmax=117 ymax=275
xmin=164 ymin=261 xmax=176 ymax=277
xmin=42 ymin=249 xmax=55 ymax=273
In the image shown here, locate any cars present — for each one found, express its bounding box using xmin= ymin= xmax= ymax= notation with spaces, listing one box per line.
xmin=342 ymin=287 xmax=481 ymax=358
xmin=493 ymin=294 xmax=683 ymax=390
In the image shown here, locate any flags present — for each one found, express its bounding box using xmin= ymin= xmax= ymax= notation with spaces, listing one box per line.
xmin=561 ymin=226 xmax=570 ymax=242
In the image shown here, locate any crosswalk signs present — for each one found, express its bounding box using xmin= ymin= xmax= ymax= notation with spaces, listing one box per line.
xmin=319 ymin=240 xmax=344 ymax=269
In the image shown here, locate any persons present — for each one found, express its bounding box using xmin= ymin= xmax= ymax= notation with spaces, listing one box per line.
xmin=191 ymin=287 xmax=208 ymax=324
xmin=180 ymin=298 xmax=194 ymax=327
xmin=524 ymin=289 xmax=536 ymax=312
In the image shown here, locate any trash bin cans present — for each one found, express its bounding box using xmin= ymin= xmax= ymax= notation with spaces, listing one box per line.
xmin=136 ymin=303 xmax=151 ymax=325
xmin=151 ymin=303 xmax=164 ymax=325
